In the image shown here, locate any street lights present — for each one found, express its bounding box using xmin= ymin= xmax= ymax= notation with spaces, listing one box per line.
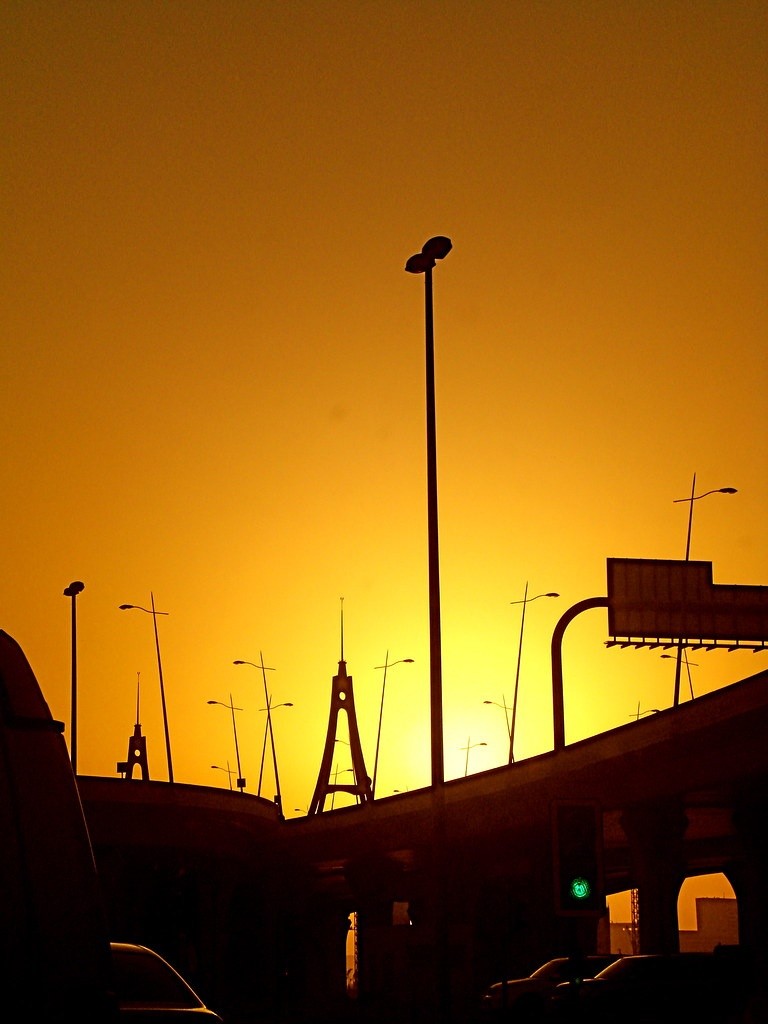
xmin=257 ymin=695 xmax=294 ymax=798
xmin=370 ymin=651 xmax=416 ymax=800
xmin=62 ymin=581 xmax=85 ymax=776
xmin=673 ymin=471 xmax=739 ymax=707
xmin=208 ymin=693 xmax=244 ymax=794
xmin=118 ymin=592 xmax=174 ymax=783
xmin=662 ymin=647 xmax=699 ymax=701
xmin=508 ymin=580 xmax=560 ymax=765
xmin=233 ymin=651 xmax=287 ymax=822
xmin=403 ymin=235 xmax=453 ymax=793
xmin=484 ymin=696 xmax=514 ymax=765
xmin=211 ymin=760 xmax=234 ymax=792
xmin=461 ymin=735 xmax=489 ymax=776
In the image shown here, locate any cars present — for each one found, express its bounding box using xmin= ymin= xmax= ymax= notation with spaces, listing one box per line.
xmin=103 ymin=940 xmax=226 ymax=1024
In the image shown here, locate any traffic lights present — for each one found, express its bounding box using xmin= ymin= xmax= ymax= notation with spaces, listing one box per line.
xmin=550 ymin=800 xmax=606 ymax=918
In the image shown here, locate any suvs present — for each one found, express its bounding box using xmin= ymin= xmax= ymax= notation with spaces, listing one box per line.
xmin=478 ymin=953 xmax=624 ymax=1024
xmin=556 ymin=953 xmax=758 ymax=1024
